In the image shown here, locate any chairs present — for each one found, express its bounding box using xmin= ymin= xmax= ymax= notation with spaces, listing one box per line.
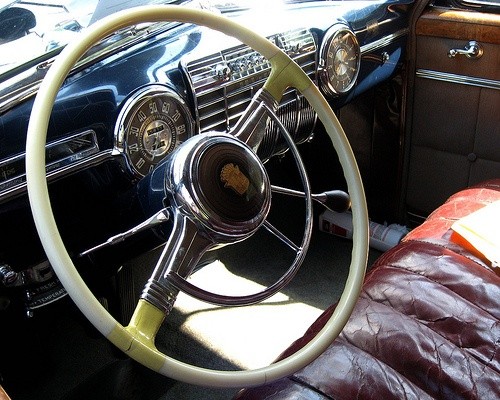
xmin=225 ymin=238 xmax=500 ymax=400
xmin=403 ymin=168 xmax=500 ymax=279
